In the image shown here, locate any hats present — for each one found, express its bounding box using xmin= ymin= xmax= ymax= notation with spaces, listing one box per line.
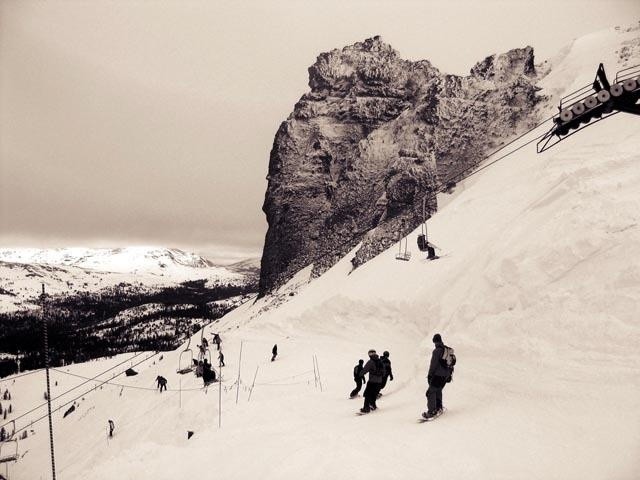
xmin=368 ymin=350 xmax=376 ymax=356
xmin=433 ymin=334 xmax=441 ymax=342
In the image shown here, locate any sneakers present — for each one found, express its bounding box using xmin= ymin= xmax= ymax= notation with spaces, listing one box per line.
xmin=422 ymin=408 xmax=442 ymax=418
xmin=361 ymin=405 xmax=377 ymax=413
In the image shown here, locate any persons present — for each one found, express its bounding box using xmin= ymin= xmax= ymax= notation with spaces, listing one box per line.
xmin=348 ymin=357 xmax=366 ymax=400
xmin=270 ymin=342 xmax=280 ymax=364
xmin=95 ymin=326 xmax=226 ymax=439
xmin=358 ymin=349 xmax=386 ymax=413
xmin=375 ymin=351 xmax=393 ymax=400
xmin=417 ymin=233 xmax=439 ymax=261
xmin=421 ymin=332 xmax=455 ymax=420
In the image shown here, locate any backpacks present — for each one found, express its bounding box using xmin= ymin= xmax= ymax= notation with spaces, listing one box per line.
xmin=440 ymin=346 xmax=456 ymax=368
xmin=371 ymin=359 xmax=382 ymax=376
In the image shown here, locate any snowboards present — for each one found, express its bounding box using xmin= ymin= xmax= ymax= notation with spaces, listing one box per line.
xmin=356 ymin=407 xmax=378 ymax=415
xmin=349 ymin=395 xmax=360 ymax=399
xmin=418 ymin=407 xmax=447 ymax=421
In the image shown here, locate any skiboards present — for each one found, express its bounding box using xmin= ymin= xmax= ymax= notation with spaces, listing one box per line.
xmin=420 ymin=254 xmax=448 ymax=264
